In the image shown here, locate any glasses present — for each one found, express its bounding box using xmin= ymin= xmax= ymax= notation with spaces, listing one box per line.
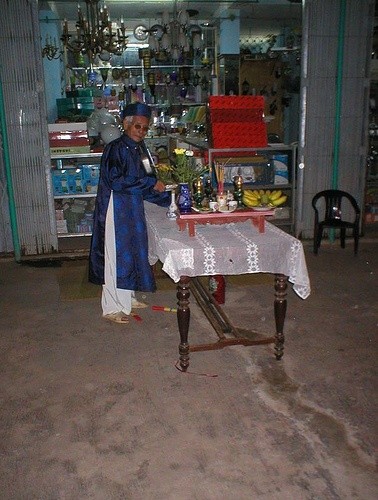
xmin=130 ymin=122 xmax=149 ymax=132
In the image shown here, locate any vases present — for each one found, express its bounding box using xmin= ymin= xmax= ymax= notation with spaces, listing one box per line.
xmin=177 ymin=183 xmax=192 ymax=212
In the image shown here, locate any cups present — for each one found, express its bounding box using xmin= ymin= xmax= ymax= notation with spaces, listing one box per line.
xmin=209 ymin=200 xmax=239 ymax=213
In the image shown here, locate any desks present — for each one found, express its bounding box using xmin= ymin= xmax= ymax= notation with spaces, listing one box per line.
xmin=143 ymin=199 xmax=311 ymax=373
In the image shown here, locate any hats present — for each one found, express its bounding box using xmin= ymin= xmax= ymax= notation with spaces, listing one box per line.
xmin=121 ymin=103 xmax=152 ymax=118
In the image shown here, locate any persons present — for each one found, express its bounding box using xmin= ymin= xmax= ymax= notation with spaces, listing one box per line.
xmin=87 ymin=103 xmax=173 ymax=324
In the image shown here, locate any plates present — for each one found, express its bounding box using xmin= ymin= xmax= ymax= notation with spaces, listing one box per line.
xmin=191 ymin=207 xmax=213 ymax=214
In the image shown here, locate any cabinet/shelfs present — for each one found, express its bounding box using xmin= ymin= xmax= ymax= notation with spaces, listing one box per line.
xmin=67 ymin=63 xmax=212 ymax=117
xmin=222 ymin=53 xmax=285 ymax=141
xmin=50 ymin=152 xmax=104 ymax=239
xmin=168 ymin=134 xmax=297 ymax=235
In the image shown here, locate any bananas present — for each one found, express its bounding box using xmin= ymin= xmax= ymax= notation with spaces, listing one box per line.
xmin=241 ymin=189 xmax=287 ymax=207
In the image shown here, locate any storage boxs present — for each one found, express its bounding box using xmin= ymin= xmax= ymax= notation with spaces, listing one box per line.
xmin=204 ymin=96 xmax=268 ymax=149
xmin=48 ymin=122 xmax=91 ymax=154
xmin=268 ymin=153 xmax=288 ymax=185
xmin=55 ymin=89 xmax=95 ymax=121
xmin=51 ymin=165 xmax=101 ymax=197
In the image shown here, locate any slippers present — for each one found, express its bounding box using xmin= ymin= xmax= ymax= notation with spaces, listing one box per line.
xmin=104 ymin=311 xmax=130 ymax=323
xmin=131 ymin=301 xmax=150 ymax=308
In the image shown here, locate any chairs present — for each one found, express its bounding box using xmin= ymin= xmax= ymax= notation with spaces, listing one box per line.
xmin=312 ymin=190 xmax=361 ymax=256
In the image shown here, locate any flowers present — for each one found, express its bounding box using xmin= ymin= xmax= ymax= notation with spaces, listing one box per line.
xmin=153 ymin=149 xmax=211 ymax=185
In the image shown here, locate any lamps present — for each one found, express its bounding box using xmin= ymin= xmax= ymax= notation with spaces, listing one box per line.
xmin=148 ymin=1 xmax=203 ymax=62
xmin=39 ymin=34 xmax=65 ymax=61
xmin=60 ymin=0 xmax=129 ymax=61
